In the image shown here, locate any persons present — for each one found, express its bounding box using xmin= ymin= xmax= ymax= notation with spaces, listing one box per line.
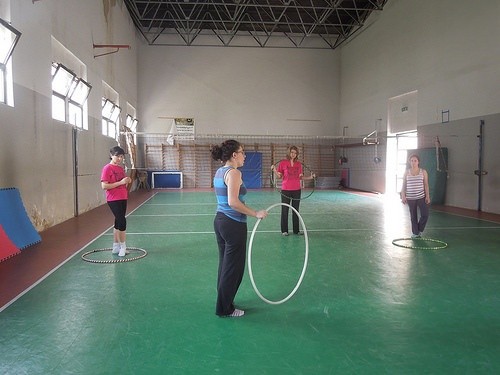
xmin=210 ymin=139 xmax=268 ymax=317
xmin=270 ymin=146 xmax=315 ymax=236
xmin=101 ymin=146 xmax=131 ymax=256
xmin=400 ymin=155 xmax=430 ymax=238
